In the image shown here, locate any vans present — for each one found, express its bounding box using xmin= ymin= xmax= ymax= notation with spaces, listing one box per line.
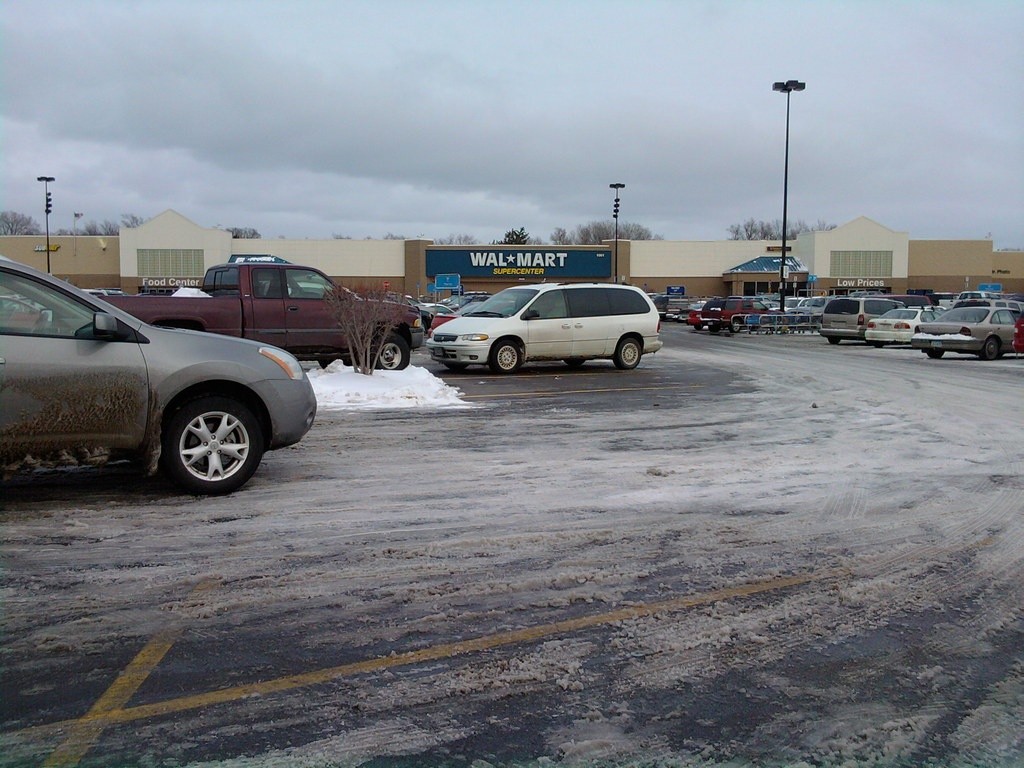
xmin=429 ymin=281 xmax=664 ymax=377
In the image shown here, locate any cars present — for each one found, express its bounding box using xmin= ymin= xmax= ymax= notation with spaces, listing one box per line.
xmin=360 ymin=288 xmax=515 ymax=338
xmin=0 ymin=259 xmax=320 ymax=499
xmin=0 ymin=288 xmax=126 ymax=332
xmin=646 ymin=285 xmax=1024 ymax=356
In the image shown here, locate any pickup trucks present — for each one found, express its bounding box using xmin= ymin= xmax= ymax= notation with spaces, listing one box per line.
xmin=50 ymin=263 xmax=428 ymax=373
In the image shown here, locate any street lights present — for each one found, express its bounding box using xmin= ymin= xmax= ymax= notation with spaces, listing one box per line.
xmin=609 ymin=183 xmax=625 ymax=284
xmin=772 ymin=80 xmax=806 ymax=313
xmin=36 ymin=176 xmax=56 ymax=275
